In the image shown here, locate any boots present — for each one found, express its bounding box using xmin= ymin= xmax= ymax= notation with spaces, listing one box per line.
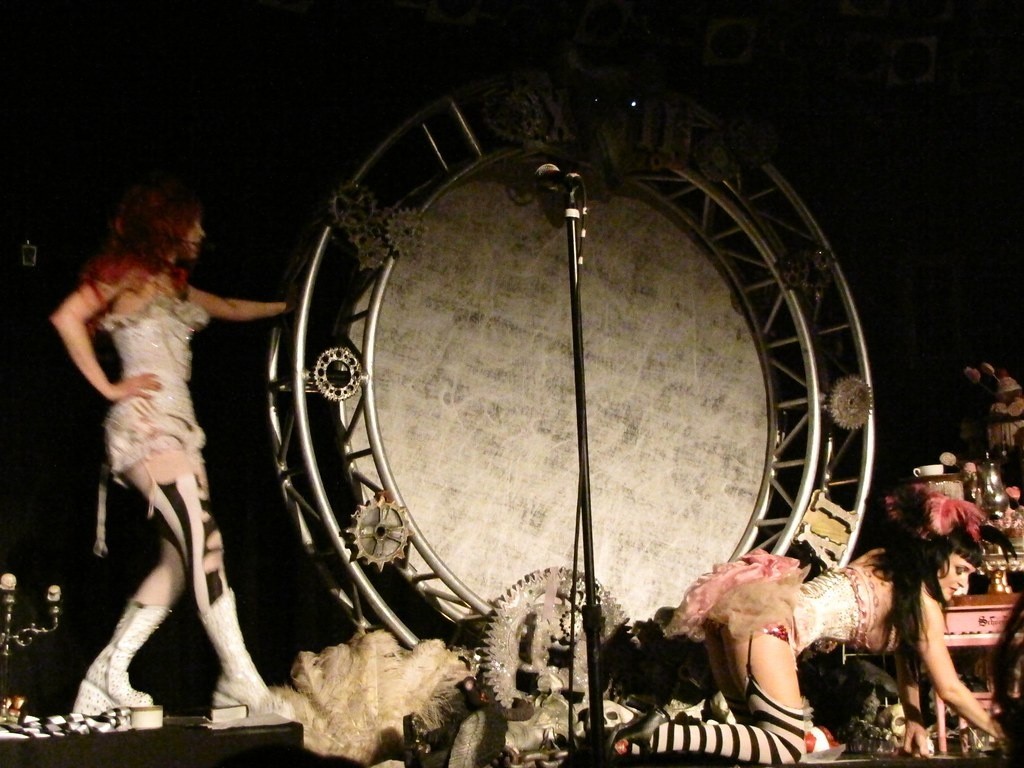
xmin=69 ymin=600 xmax=170 ymax=719
xmin=197 ymin=588 xmax=295 ymax=720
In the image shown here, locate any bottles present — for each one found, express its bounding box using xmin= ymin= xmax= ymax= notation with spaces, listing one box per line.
xmin=975 ymin=450 xmax=1011 ymax=521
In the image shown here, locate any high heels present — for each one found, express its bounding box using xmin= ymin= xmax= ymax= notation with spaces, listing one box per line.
xmin=602 ymin=695 xmax=668 ymax=762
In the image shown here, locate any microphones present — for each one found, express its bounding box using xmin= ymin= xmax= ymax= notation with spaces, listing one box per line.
xmin=535 ymin=164 xmax=582 ymax=193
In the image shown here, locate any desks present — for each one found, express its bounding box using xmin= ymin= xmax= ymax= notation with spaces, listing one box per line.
xmin=914 ymin=605 xmax=1024 ymax=751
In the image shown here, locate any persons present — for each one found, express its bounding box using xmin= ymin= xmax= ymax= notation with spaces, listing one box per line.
xmin=597 ymin=483 xmax=1008 ymax=766
xmin=49 ymin=182 xmax=304 ymax=728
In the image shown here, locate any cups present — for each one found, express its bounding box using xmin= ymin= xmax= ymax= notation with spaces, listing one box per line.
xmin=913 ymin=464 xmax=945 ymax=478
